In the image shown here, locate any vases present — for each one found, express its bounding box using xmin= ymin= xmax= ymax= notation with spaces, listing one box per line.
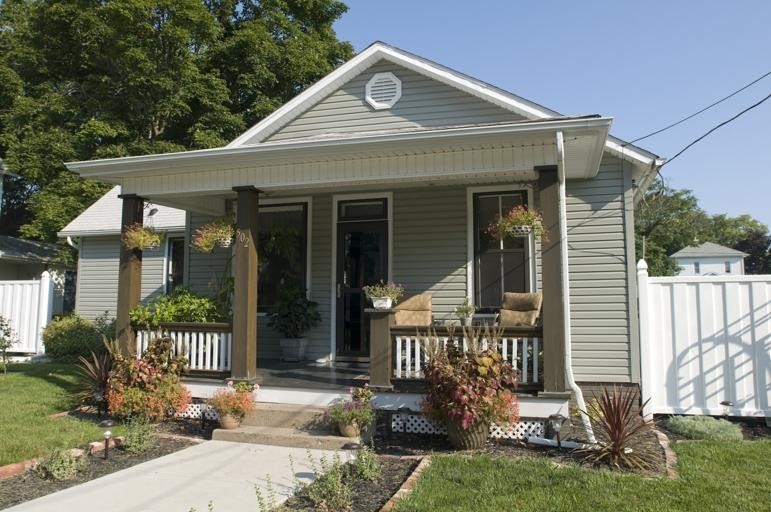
xmin=447 ymin=416 xmax=488 ymax=450
xmin=338 ymin=418 xmax=362 ymax=436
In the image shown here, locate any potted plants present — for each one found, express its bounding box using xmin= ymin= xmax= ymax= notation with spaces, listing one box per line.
xmin=209 ymin=389 xmax=253 ymax=429
xmin=486 ymin=205 xmax=551 ymax=243
xmin=362 ymin=278 xmax=405 ymax=310
xmin=121 ymin=216 xmax=236 ymax=252
xmin=453 ymin=297 xmax=478 ymax=326
xmin=266 ymin=286 xmax=321 ymax=362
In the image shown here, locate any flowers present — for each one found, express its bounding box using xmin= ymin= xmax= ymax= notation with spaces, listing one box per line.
xmin=418 ymin=340 xmax=522 ymax=430
xmin=330 ymin=383 xmax=373 ymax=431
xmin=227 ymin=379 xmax=260 ymax=393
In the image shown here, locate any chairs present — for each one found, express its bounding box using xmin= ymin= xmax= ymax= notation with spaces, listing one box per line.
xmin=393 ymin=293 xmax=432 ymax=372
xmin=495 ymin=290 xmax=542 ymax=366
